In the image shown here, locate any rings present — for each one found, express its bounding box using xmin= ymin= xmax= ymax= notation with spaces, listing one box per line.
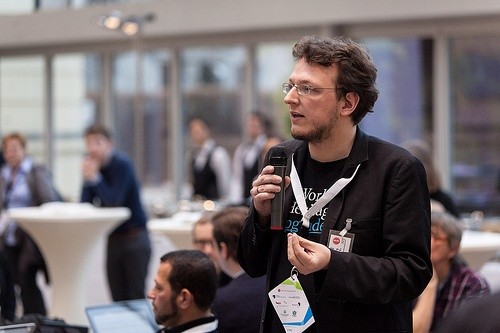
xmin=256 ymin=186 xmax=260 ymax=193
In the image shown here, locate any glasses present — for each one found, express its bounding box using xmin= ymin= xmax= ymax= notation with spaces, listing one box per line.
xmin=281 ymin=83 xmax=347 ymax=95
xmin=193 ymin=238 xmax=216 ymax=251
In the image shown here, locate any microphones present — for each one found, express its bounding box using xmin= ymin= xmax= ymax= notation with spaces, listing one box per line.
xmin=268 ymin=145 xmax=288 ymax=230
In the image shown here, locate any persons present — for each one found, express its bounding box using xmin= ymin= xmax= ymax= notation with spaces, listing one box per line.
xmin=187 ymin=117 xmax=232 ymax=200
xmin=236 ymin=33 xmax=434 ymax=333
xmin=79 ymin=124 xmax=152 ymax=303
xmin=428 ymin=210 xmax=491 ymax=328
xmin=405 ymin=268 xmax=500 ymax=333
xmin=146 ymin=204 xmax=267 ymax=332
xmin=0 ymin=133 xmax=63 ymax=322
xmin=398 ymin=143 xmax=461 ymax=219
xmin=234 ymin=110 xmax=282 ymax=205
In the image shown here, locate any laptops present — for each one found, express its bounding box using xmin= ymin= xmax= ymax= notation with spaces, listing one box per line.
xmin=86 ymin=298 xmax=160 ymax=333
